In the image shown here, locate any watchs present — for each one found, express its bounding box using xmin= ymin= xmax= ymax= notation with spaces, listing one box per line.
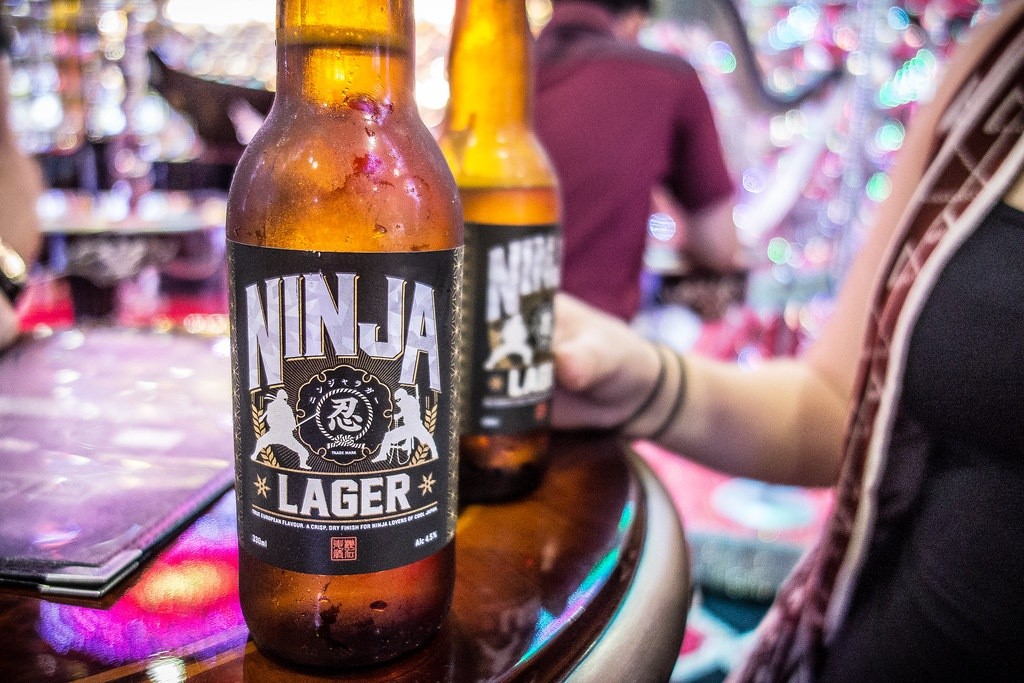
xmin=0 ymin=243 xmax=32 ymax=310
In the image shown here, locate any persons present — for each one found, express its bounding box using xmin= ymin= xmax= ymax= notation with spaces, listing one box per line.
xmin=0 ymin=53 xmax=42 ymax=352
xmin=548 ymin=0 xmax=1024 ymax=681
xmin=526 ymin=1 xmax=734 ymax=323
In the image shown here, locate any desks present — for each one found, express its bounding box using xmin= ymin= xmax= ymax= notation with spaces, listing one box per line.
xmin=0 ymin=346 xmax=691 ymax=683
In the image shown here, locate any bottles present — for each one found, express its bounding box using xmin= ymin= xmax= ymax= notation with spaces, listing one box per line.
xmin=226 ymin=1 xmax=467 ymax=675
xmin=418 ymin=2 xmax=565 ymax=499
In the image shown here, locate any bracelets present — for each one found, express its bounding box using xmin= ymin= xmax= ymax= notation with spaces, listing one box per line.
xmin=613 ymin=337 xmax=686 ymax=443
